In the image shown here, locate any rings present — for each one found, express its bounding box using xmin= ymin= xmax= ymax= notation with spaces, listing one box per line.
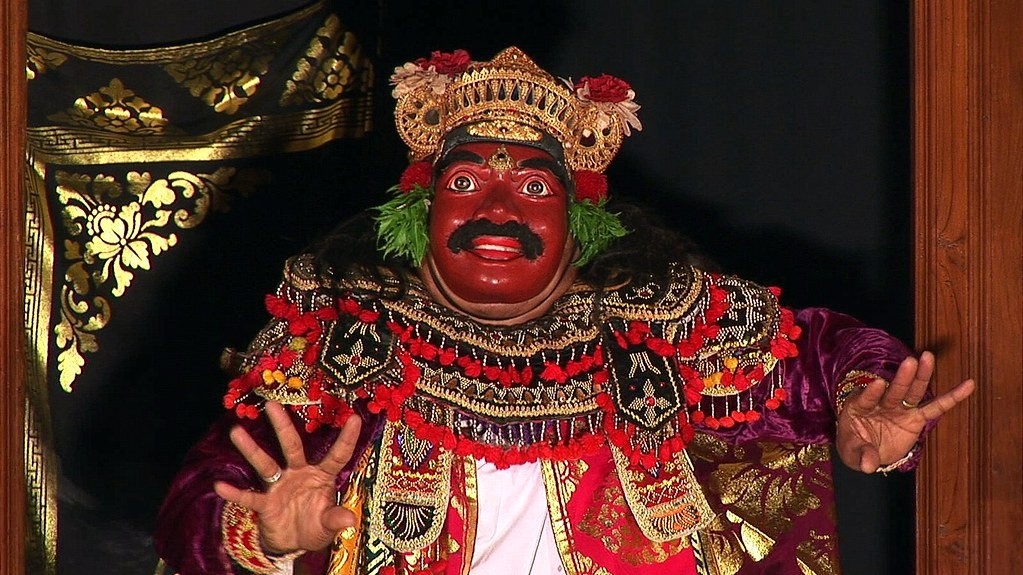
xmin=902 ymin=400 xmax=918 ymax=408
xmin=262 ymin=466 xmax=282 ymax=483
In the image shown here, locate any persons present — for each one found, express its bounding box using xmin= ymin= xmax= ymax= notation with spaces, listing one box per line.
xmin=154 ymin=47 xmax=976 ymax=575
